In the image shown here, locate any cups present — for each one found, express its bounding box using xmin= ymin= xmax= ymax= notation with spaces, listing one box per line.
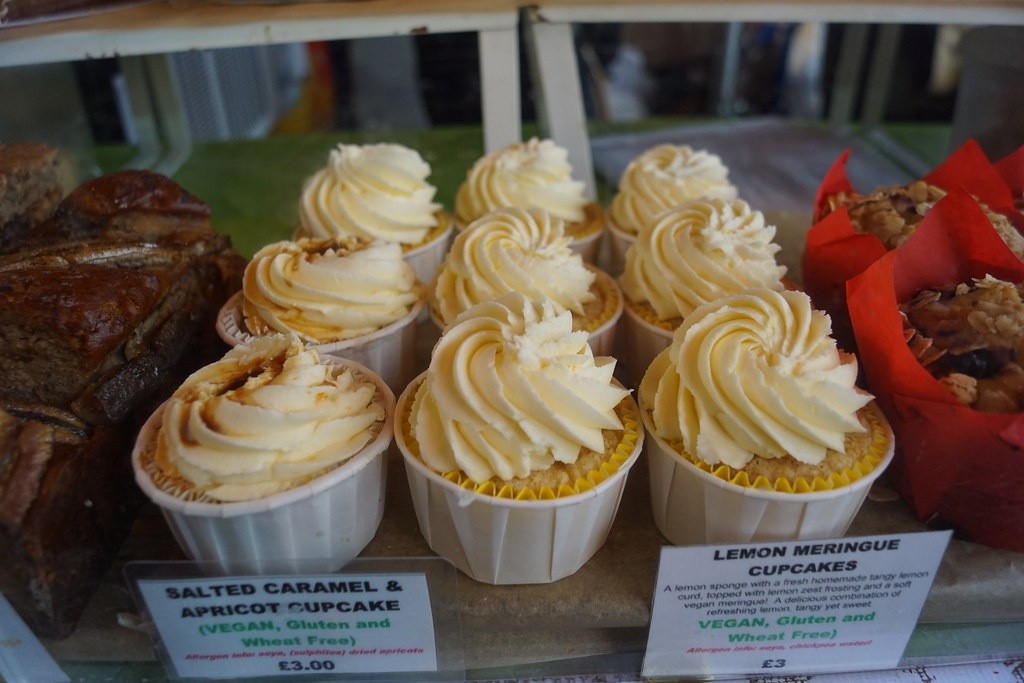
xmin=391 ymin=369 xmax=646 ymax=586
xmin=638 ymin=348 xmax=895 ymax=546
xmin=291 ymin=207 xmax=790 ymax=367
xmin=209 ymin=266 xmax=428 ymax=412
xmin=123 ymin=358 xmax=392 ymax=581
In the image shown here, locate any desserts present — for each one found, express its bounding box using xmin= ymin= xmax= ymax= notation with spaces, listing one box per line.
xmin=131 ymin=137 xmax=894 ymax=587
xmin=800 ymin=138 xmax=1023 ymax=554
